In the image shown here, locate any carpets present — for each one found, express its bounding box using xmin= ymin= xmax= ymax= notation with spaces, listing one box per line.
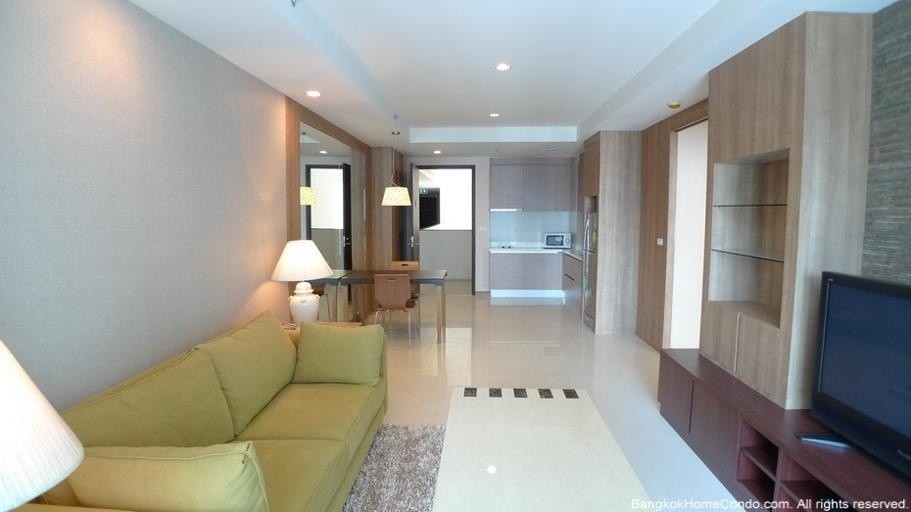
xmin=341 ymin=420 xmax=448 ymax=511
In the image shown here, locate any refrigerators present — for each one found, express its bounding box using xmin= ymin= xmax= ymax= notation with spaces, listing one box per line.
xmin=581 ymin=212 xmax=598 ymax=334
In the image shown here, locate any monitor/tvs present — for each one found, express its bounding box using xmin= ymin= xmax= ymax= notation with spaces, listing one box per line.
xmin=796 ymin=270 xmax=911 ymax=482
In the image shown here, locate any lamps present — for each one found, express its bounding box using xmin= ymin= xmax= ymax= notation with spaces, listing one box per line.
xmin=270 ymin=239 xmax=335 ymax=331
xmin=300 ymin=131 xmax=319 ymax=206
xmin=0 ymin=336 xmax=87 ymax=512
xmin=381 ymin=130 xmax=413 ymax=207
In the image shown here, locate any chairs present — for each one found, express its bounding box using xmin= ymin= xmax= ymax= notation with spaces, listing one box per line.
xmin=384 ymin=259 xmax=423 ymax=326
xmin=372 ymin=272 xmax=422 ymax=348
xmin=309 ymin=283 xmax=333 ymax=323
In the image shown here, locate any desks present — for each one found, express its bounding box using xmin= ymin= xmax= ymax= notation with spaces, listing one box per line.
xmin=282 ymin=321 xmax=363 ymax=333
xmin=303 ymin=268 xmax=358 ymax=323
xmin=341 ymin=269 xmax=450 ymax=345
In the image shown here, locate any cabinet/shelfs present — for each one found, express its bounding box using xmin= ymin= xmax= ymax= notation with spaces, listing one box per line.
xmin=699 ymin=10 xmax=875 ymax=411
xmin=488 ymin=157 xmax=524 ymax=212
xmin=582 ymin=131 xmax=641 ymax=335
xmin=657 ymin=345 xmax=911 ymax=511
xmin=523 ymin=157 xmax=578 ymax=212
xmin=563 ymin=252 xmax=584 ymax=318
xmin=489 ymin=252 xmax=565 ymax=299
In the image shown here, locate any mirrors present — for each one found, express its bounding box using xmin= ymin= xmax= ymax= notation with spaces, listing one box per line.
xmin=285 ymin=94 xmax=376 ymax=324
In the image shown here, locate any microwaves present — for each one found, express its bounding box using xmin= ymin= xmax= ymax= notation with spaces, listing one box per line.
xmin=543 ymin=232 xmax=572 ymax=249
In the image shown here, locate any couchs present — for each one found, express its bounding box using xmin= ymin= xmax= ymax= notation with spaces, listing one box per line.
xmin=11 ymin=309 xmax=391 ymax=512
